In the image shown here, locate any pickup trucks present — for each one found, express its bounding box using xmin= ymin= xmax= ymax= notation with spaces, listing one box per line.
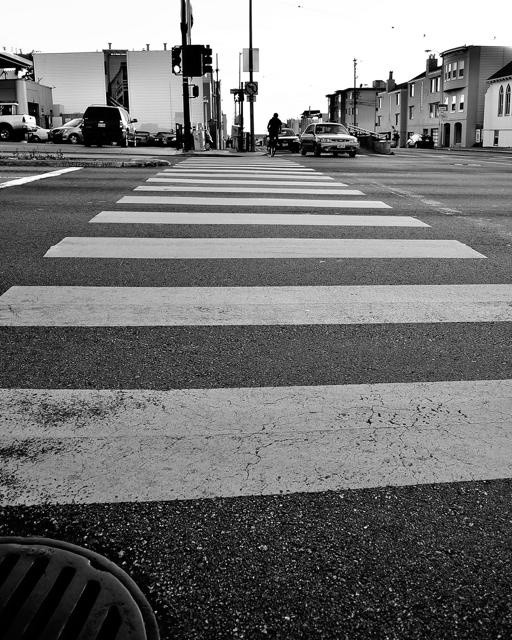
xmin=0 ymin=113 xmax=36 ymax=144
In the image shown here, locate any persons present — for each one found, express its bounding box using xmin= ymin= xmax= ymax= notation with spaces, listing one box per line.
xmin=267 ymin=113 xmax=282 ymax=146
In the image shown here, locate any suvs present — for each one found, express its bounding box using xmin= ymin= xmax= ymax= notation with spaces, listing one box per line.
xmin=80 ymin=105 xmax=140 ymax=149
xmin=50 ymin=117 xmax=84 ymax=145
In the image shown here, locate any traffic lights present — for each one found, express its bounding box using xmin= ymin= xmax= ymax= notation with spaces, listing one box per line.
xmin=193 ymin=86 xmax=199 ymax=97
xmin=234 ymin=92 xmax=244 ymax=102
xmin=199 ymin=45 xmax=214 ymax=75
xmin=171 ymin=46 xmax=182 ymax=75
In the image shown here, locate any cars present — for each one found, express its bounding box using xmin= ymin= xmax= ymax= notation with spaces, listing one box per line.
xmin=254 ymin=122 xmax=360 ymax=159
xmin=25 ymin=124 xmax=214 ymax=151
xmin=406 ymin=132 xmax=436 ymax=150
